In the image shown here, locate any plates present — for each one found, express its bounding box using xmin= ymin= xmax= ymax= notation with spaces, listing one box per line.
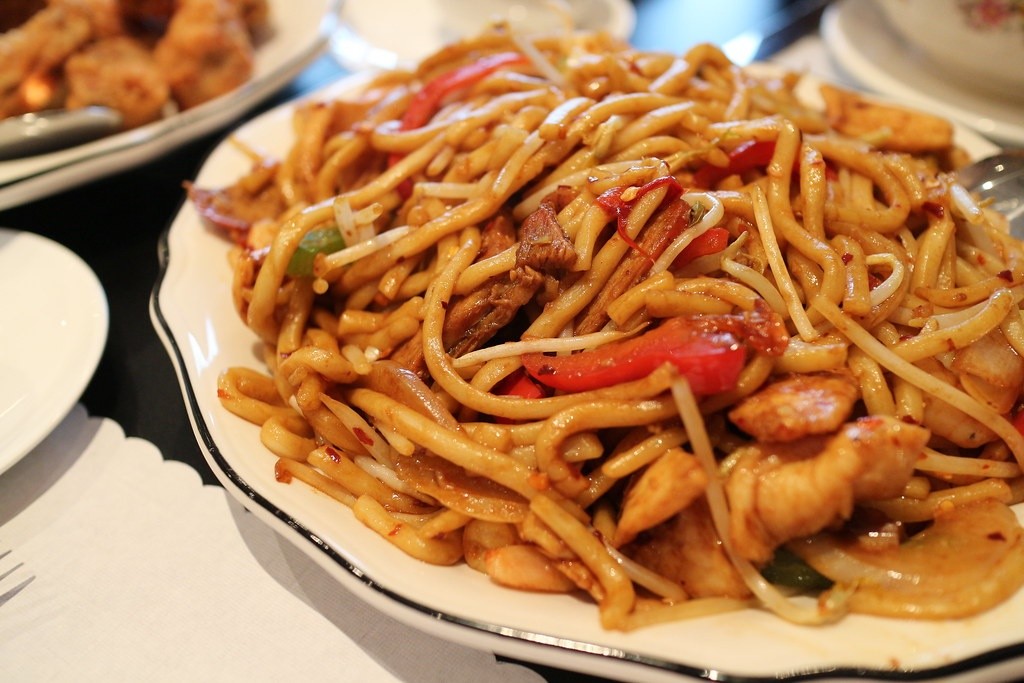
xmin=147 ymin=76 xmax=1024 ymax=683
xmin=820 ymin=0 xmax=1024 ymax=145
xmin=0 ymin=227 xmax=108 ymax=477
xmin=0 ymin=0 xmax=342 ymax=212
xmin=326 ymin=0 xmax=637 ymax=75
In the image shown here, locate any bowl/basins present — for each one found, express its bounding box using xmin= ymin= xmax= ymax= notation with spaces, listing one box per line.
xmin=874 ymin=0 xmax=1024 ymax=108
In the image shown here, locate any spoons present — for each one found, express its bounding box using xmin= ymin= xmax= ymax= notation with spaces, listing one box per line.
xmin=954 ymin=152 xmax=1024 ymax=240
xmin=0 ymin=105 xmax=125 ymax=162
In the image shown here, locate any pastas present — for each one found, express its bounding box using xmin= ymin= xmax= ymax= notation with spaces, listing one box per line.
xmin=185 ymin=15 xmax=1024 ymax=637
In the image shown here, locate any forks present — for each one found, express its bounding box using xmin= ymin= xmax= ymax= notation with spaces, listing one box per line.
xmin=0 ymin=549 xmax=37 ymax=608
